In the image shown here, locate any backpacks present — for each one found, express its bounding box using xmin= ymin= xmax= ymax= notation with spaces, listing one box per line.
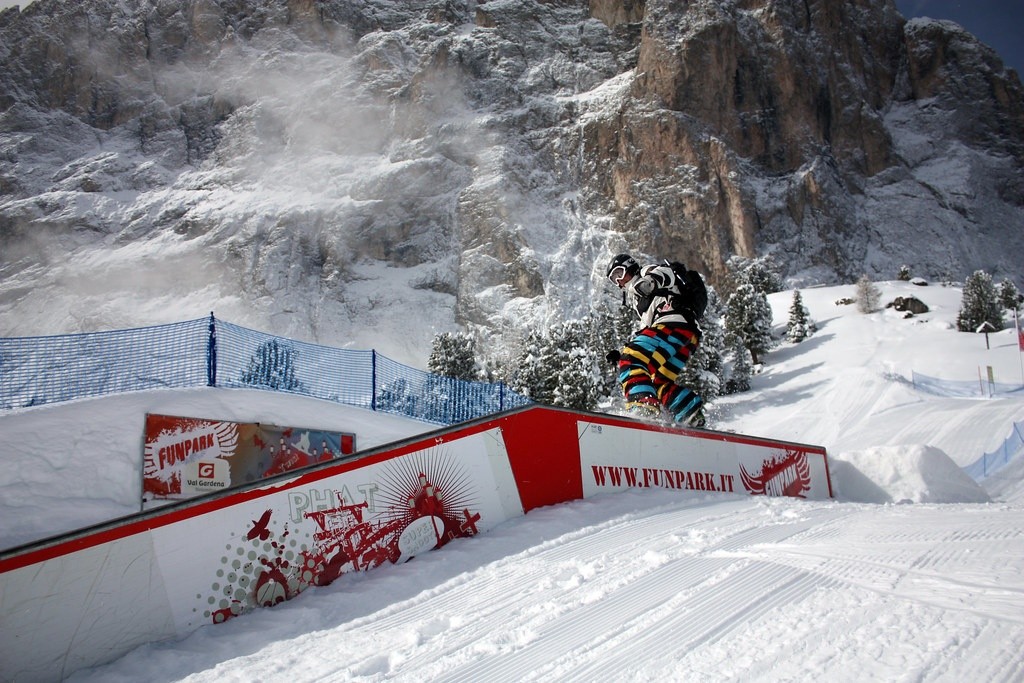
xmin=645 ymin=261 xmax=708 ymax=318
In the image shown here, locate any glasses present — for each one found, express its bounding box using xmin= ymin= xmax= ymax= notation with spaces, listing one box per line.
xmin=608 ymin=265 xmax=627 ymax=287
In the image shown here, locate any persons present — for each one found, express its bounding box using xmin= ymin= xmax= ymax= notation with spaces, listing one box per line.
xmin=606 ymin=254 xmax=707 ymax=428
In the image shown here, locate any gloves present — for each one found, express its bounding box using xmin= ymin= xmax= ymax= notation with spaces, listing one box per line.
xmin=635 ymin=276 xmax=655 ymax=296
xmin=606 ymin=350 xmax=621 ymax=365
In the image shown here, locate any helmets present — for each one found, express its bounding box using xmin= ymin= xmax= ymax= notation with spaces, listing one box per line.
xmin=607 ymin=253 xmax=640 ymax=275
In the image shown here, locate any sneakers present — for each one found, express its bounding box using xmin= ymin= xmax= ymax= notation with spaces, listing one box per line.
xmin=682 ymin=408 xmax=707 ymax=429
